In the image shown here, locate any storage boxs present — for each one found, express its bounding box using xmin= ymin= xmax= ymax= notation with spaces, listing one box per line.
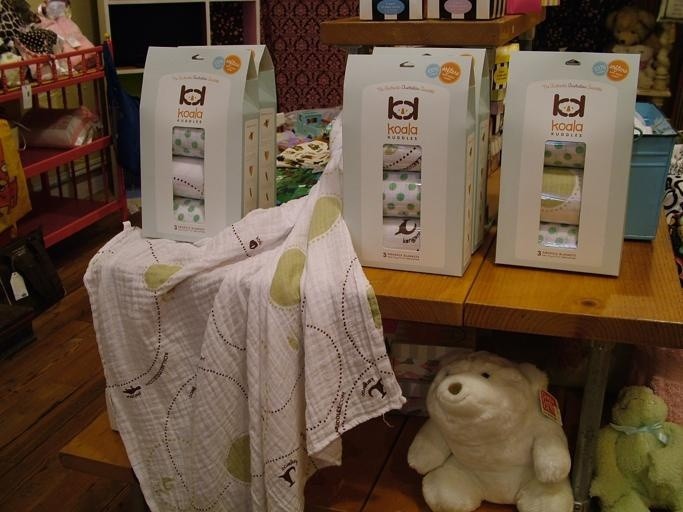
xmin=626 ymin=102 xmax=677 ymax=240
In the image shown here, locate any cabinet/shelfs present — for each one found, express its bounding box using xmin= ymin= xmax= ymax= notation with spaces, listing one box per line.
xmin=0 ymin=38 xmax=127 ymax=250
xmin=61 ymin=10 xmax=682 ymax=511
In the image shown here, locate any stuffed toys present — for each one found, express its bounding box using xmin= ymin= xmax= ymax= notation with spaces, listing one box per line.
xmin=407 ymin=350 xmax=574 ymax=512
xmin=589 ymin=386 xmax=683 ymax=512
